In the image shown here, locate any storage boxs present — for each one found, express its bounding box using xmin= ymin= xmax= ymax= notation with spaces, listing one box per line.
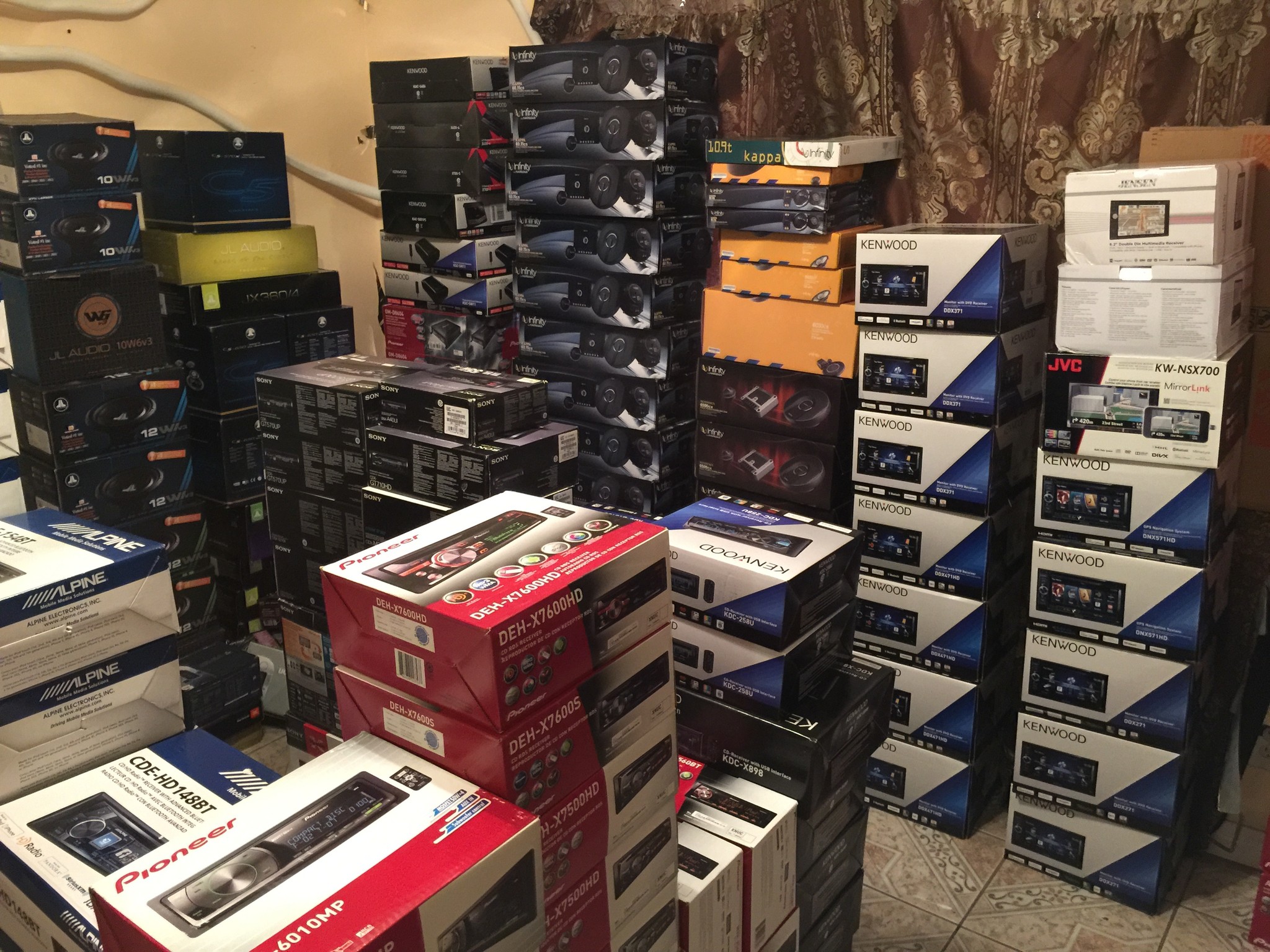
xmin=0 ymin=26 xmax=1270 ymax=952
